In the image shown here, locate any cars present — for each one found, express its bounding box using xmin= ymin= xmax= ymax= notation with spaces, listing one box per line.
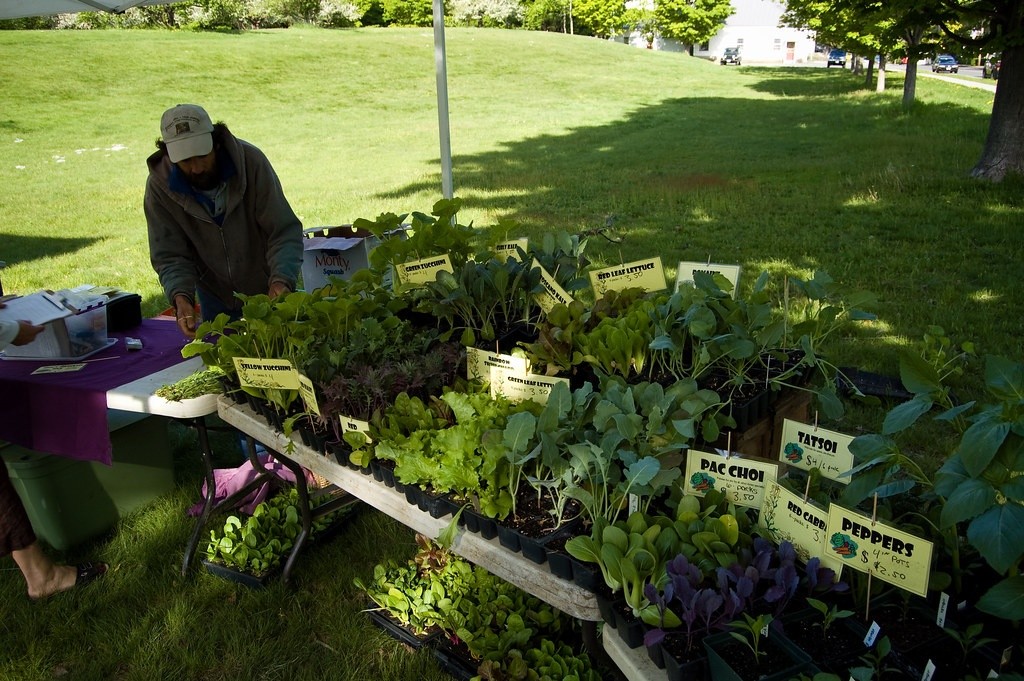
xmin=720 ymin=47 xmax=742 ymax=65
xmin=932 ymin=55 xmax=959 ymax=73
xmin=826 ymin=49 xmax=847 ymax=68
xmin=990 ymin=60 xmax=1001 ymax=80
xmin=983 ymin=61 xmax=994 ymax=79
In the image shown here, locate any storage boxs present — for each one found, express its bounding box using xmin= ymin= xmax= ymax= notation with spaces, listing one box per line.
xmin=302 ymin=225 xmax=412 ymax=293
xmin=4 ymin=286 xmax=142 ymax=358
xmin=1 ymin=408 xmax=178 ymax=552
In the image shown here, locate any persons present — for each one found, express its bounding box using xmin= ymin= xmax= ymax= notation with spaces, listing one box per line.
xmin=0 ymin=277 xmax=108 ymax=602
xmin=145 ymin=105 xmax=304 ymax=479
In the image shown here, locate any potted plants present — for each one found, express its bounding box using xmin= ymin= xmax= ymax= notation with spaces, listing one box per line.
xmin=179 ymin=197 xmax=1024 ymax=681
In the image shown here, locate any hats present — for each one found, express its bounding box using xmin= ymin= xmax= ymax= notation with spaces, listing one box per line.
xmin=160 ymin=104 xmax=213 ymax=163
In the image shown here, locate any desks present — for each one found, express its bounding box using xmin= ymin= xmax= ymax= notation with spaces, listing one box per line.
xmin=0 ymin=319 xmax=288 ymax=581
xmin=218 ymin=392 xmax=605 ymax=681
xmin=603 ymin=623 xmax=670 ymax=681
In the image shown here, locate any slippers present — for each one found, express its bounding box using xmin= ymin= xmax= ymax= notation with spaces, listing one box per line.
xmin=32 ymin=562 xmax=109 ymax=602
